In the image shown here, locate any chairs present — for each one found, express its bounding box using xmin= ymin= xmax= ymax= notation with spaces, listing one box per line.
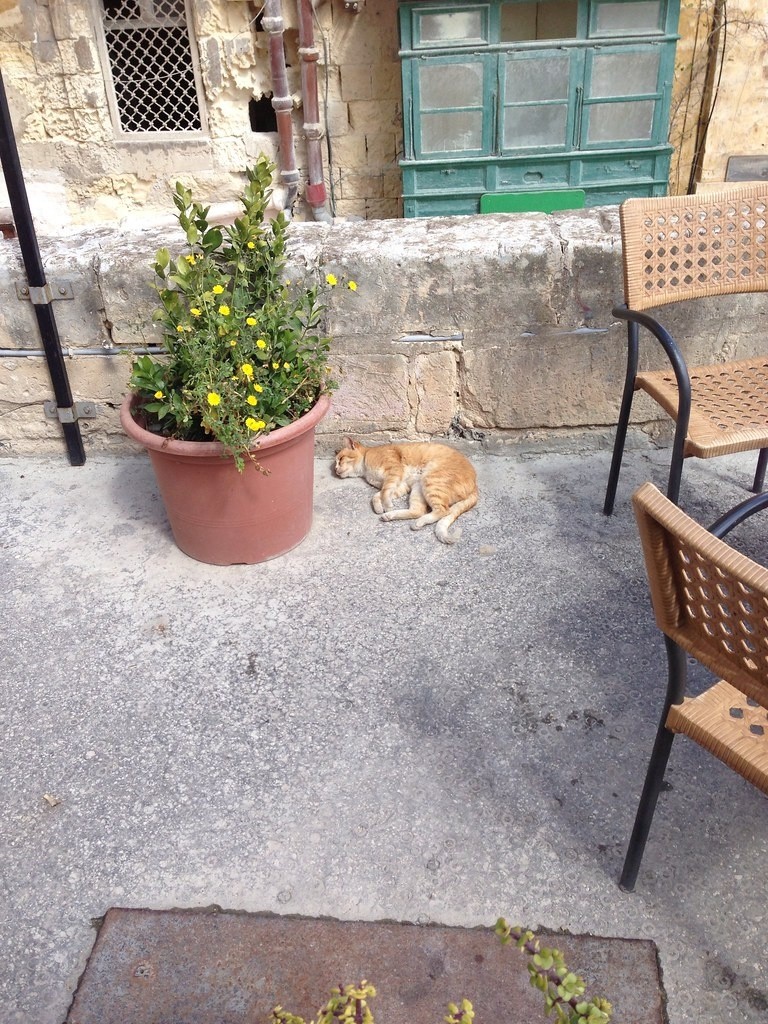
xmin=603 ymin=183 xmax=768 ymax=516
xmin=619 ymin=481 xmax=768 ymax=893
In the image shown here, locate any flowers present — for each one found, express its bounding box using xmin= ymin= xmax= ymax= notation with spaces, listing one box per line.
xmin=117 ymin=149 xmax=359 ymax=476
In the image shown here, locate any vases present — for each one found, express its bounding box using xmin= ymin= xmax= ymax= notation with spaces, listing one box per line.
xmin=117 ymin=361 xmax=331 ymax=565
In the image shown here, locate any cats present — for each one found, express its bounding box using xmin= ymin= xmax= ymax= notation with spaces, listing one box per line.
xmin=334 ymin=435 xmax=479 ymax=545
